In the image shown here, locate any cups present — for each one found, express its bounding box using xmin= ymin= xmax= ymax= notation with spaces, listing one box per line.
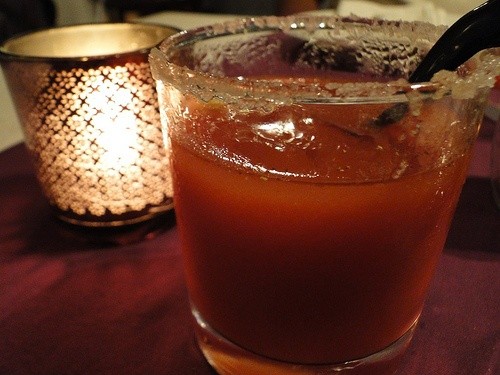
xmin=146 ymin=15 xmax=500 ymax=374
xmin=0 ymin=22 xmax=187 ymax=228
